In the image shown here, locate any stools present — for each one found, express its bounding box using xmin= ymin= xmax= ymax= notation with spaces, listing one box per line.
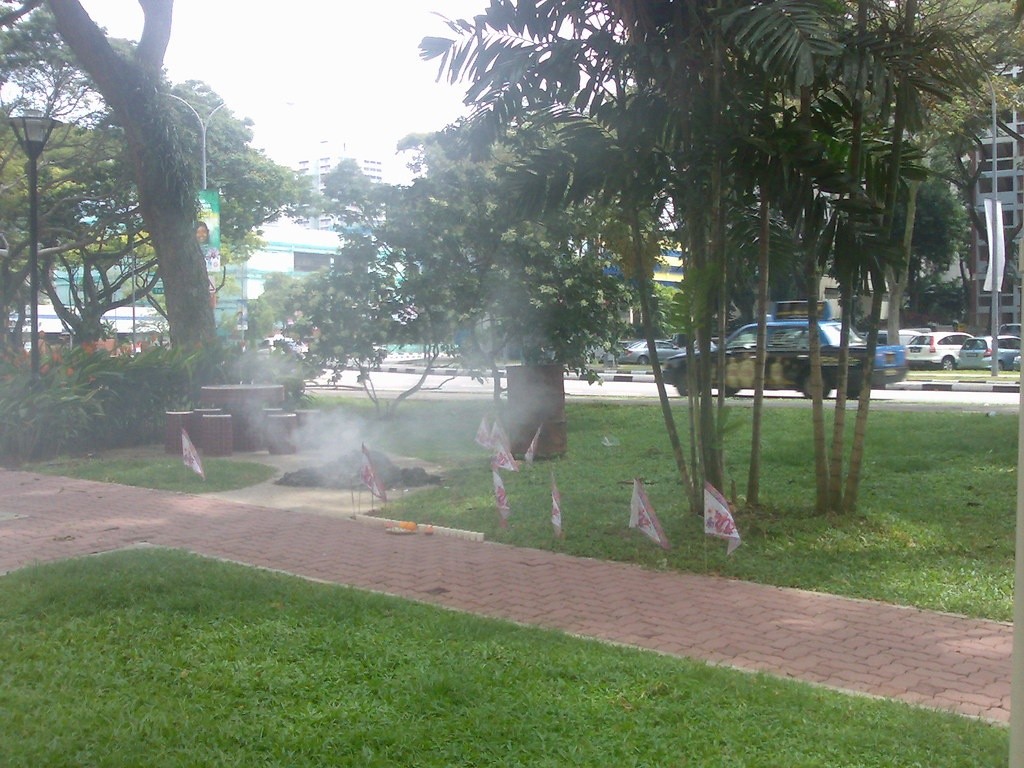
xmin=165 ymin=408 xmax=323 ymax=457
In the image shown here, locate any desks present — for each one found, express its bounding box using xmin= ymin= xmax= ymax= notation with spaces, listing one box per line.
xmin=202 ymin=383 xmax=284 ymax=453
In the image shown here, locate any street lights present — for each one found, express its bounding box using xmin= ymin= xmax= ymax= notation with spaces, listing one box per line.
xmin=162 ymin=86 xmax=250 ymax=183
xmin=4 ymin=106 xmax=66 ymax=386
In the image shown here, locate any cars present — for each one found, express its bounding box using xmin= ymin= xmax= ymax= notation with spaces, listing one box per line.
xmin=902 ymin=331 xmax=976 ymax=372
xmin=1000 ymin=322 xmax=1023 ymax=338
xmin=956 ymin=336 xmax=1022 ymax=371
xmin=653 ymin=297 xmax=909 ymax=402
xmin=1012 ymin=352 xmax=1022 ymax=374
xmin=864 ymin=329 xmax=925 ymax=351
xmin=618 ymin=340 xmax=685 ymax=365
